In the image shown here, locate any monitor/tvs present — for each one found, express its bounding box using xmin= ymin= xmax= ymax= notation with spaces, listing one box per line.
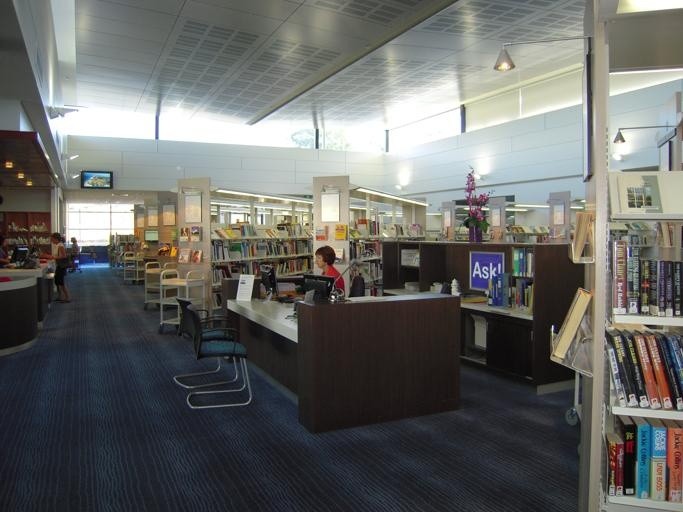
xmin=10 ymin=245 xmax=29 ymax=263
xmin=80 ymin=170 xmax=113 ymax=189
xmin=303 ymin=274 xmax=335 ymax=301
xmin=259 ymin=263 xmax=281 ymax=298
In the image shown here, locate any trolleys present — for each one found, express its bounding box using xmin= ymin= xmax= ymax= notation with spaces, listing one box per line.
xmin=123 ymin=251 xmax=146 ymax=284
xmin=158 ymin=269 xmax=208 ymax=338
xmin=143 ymin=261 xmax=178 ymax=310
xmin=563 ymin=372 xmax=582 ymax=457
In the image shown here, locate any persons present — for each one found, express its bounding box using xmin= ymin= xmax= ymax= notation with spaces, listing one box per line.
xmin=0 ymin=237 xmax=10 ymax=268
xmin=70 ymin=237 xmax=80 ymax=272
xmin=46 ymin=232 xmax=71 ymax=302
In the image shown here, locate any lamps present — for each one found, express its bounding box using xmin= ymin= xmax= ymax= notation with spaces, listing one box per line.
xmin=613 ymin=123 xmax=673 ymax=144
xmin=492 ymin=35 xmax=589 ymax=72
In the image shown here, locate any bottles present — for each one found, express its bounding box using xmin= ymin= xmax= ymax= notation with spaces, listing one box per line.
xmin=450 ymin=278 xmax=460 ymax=297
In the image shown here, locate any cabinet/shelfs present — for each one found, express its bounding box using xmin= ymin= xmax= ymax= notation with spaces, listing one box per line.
xmin=576 ymin=2 xmax=682 ymax=511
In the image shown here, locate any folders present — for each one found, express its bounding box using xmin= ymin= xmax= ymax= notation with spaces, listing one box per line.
xmin=512 ymin=246 xmax=533 ymax=278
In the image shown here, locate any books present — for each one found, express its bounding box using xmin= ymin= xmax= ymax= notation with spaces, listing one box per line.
xmin=599 ymin=219 xmax=682 ymax=505
xmin=551 ymin=287 xmax=592 ymax=359
xmin=194 ymin=211 xmax=550 ymax=316
xmin=570 ymin=212 xmax=592 ymax=261
xmin=157 ymin=225 xmax=201 ymax=266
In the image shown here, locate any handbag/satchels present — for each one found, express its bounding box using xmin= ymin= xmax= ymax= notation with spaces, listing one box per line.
xmin=56 ymin=259 xmax=67 ymax=267
